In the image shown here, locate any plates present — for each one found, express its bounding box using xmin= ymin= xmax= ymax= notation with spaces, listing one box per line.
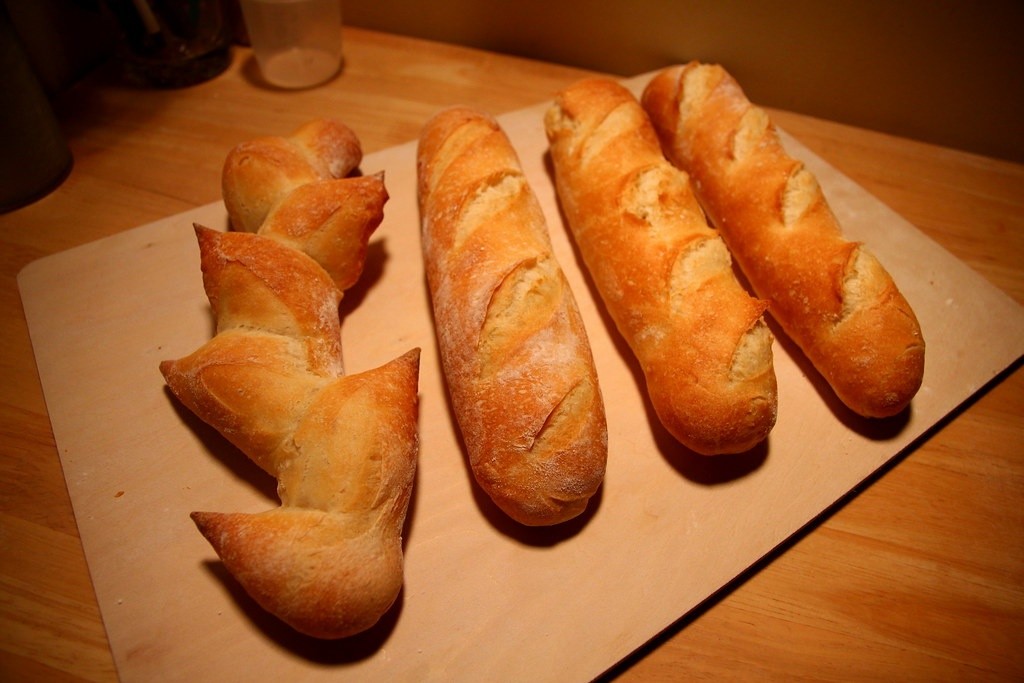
xmin=17 ymin=64 xmax=1024 ymax=683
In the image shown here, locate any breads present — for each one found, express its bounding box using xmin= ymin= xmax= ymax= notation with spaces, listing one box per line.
xmin=417 ymin=107 xmax=608 ymax=527
xmin=639 ymin=60 xmax=925 ymax=419
xmin=157 ymin=118 xmax=422 ymax=641
xmin=542 ymin=76 xmax=778 ymax=456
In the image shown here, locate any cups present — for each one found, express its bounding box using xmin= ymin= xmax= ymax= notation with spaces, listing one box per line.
xmin=103 ymin=0 xmax=234 ymax=89
xmin=241 ymin=0 xmax=346 ymax=90
xmin=0 ymin=0 xmax=75 ymax=215
